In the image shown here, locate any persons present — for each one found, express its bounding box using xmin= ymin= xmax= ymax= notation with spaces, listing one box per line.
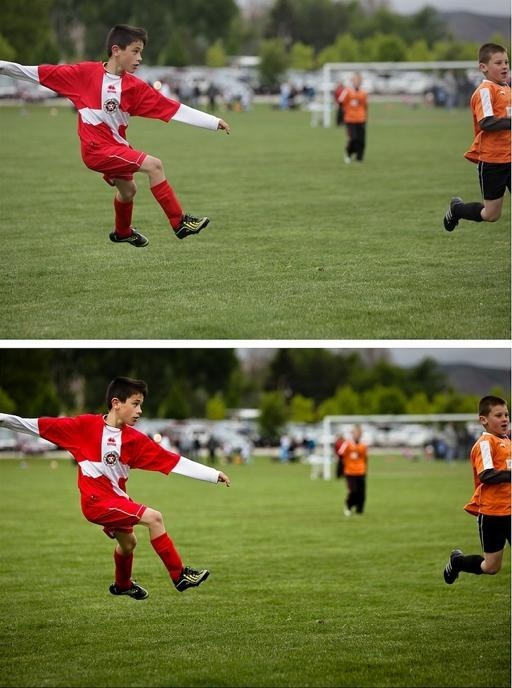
xmin=440 ymin=43 xmax=511 ymax=231
xmin=275 ymin=75 xmax=318 ymax=111
xmin=0 ymin=414 xmax=253 ymax=469
xmin=1 ymin=375 xmax=230 ymax=599
xmin=420 ymin=426 xmax=480 ymax=462
xmin=278 ymin=433 xmax=318 ymax=468
xmin=1 ymin=20 xmax=232 ymax=248
xmin=425 ymin=72 xmax=480 ymax=109
xmin=442 ymin=394 xmax=512 ymax=585
xmin=335 ymin=432 xmax=347 ymax=478
xmin=335 ymin=70 xmax=368 ymax=162
xmin=1 ymin=64 xmax=254 ymax=114
xmin=337 ymin=421 xmax=368 ymax=517
xmin=336 ymin=83 xmax=347 ymax=125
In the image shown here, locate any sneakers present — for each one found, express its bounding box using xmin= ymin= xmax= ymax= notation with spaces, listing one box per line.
xmin=343 ymin=503 xmax=353 ymax=518
xmin=445 ymin=549 xmax=463 ymax=584
xmin=109 ymin=580 xmax=149 ymax=600
xmin=172 ymin=215 xmax=209 ymax=239
xmin=109 ymin=229 xmax=150 ymax=247
xmin=344 ymin=148 xmax=352 ymax=165
xmin=174 ymin=566 xmax=210 ymax=592
xmin=444 ymin=196 xmax=463 ymax=232
